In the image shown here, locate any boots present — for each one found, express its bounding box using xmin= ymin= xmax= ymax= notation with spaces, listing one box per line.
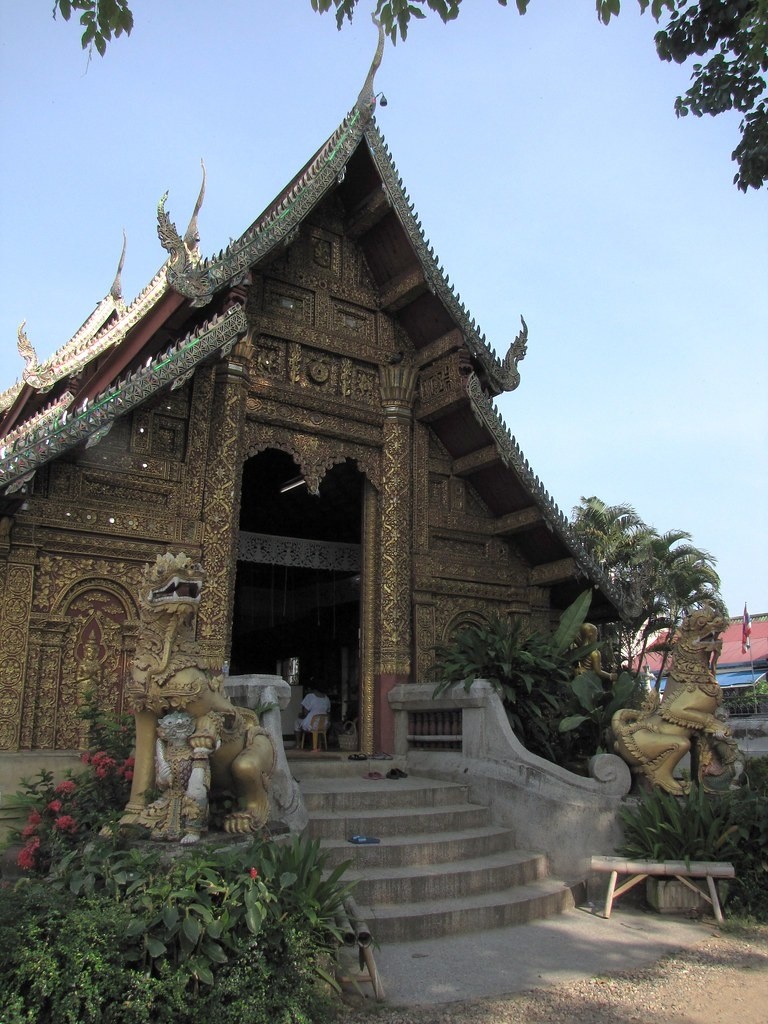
xmin=293 ymin=730 xmax=302 ymax=749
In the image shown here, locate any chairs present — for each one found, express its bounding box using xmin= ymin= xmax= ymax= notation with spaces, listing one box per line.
xmin=301 ymin=714 xmax=331 ymax=750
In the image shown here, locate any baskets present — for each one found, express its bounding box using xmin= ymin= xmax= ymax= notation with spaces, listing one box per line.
xmin=337 ymin=721 xmax=358 ymax=750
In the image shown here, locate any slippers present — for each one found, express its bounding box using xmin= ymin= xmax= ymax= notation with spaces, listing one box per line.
xmin=349 ymin=753 xmax=367 ymax=761
xmin=368 ymin=751 xmax=392 ymax=760
xmin=374 ymin=771 xmax=385 ymax=779
xmin=348 ymin=835 xmax=381 ymax=844
xmin=386 ymin=769 xmax=398 ymax=779
xmin=395 ymin=767 xmax=407 ymax=778
xmin=364 ymin=773 xmax=378 ymax=780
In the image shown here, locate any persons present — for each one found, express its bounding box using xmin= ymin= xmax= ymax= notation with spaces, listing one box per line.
xmin=571 ymin=624 xmax=617 ymax=760
xmin=293 ymin=676 xmax=330 ymax=750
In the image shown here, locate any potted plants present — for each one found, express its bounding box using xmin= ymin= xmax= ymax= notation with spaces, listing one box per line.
xmin=615 ymin=779 xmax=748 ymax=911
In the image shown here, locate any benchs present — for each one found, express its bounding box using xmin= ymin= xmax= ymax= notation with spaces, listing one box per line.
xmin=589 ymin=855 xmax=736 ymax=923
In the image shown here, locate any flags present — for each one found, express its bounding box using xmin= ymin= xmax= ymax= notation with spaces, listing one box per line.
xmin=742 ymin=607 xmax=751 ymax=655
